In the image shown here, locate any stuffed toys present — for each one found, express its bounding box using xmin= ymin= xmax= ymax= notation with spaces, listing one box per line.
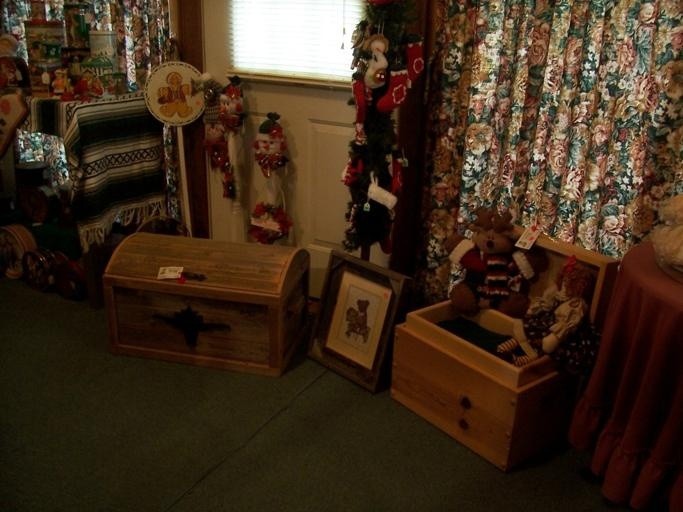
xmin=249 ymin=203 xmax=291 ymax=244
xmin=218 ymin=76 xmax=246 ymax=130
xmin=445 ymin=208 xmax=549 ymax=317
xmin=253 ymin=113 xmax=289 ymax=176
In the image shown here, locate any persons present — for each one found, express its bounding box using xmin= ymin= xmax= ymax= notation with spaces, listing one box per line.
xmin=496 ymin=258 xmax=589 ymax=368
xmin=158 ymin=72 xmax=193 ymax=117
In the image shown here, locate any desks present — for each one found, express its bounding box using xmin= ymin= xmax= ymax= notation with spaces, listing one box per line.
xmin=566 ymin=239 xmax=683 ymax=512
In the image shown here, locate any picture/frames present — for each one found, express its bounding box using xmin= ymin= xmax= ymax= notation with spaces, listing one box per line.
xmin=306 ymin=248 xmax=414 ymax=395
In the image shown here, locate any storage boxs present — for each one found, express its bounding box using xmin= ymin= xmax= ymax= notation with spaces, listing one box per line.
xmin=390 ymin=222 xmax=621 ymax=475
xmin=103 ymin=231 xmax=311 ymax=379
